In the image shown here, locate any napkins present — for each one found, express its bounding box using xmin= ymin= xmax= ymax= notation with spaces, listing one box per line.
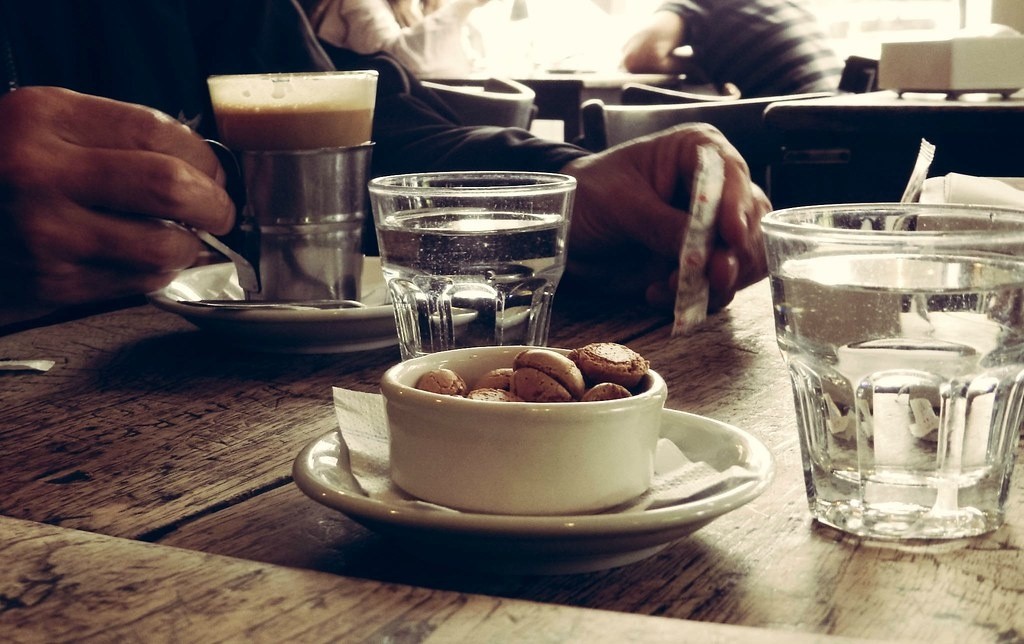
xmin=330 ymin=382 xmax=762 ymax=511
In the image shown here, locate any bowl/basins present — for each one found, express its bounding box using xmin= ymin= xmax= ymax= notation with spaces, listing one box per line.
xmin=380 ymin=345 xmax=667 ymax=515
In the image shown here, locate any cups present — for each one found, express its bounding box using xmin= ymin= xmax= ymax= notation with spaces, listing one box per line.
xmin=181 ymin=138 xmax=377 ymax=313
xmin=367 ymin=171 xmax=578 ymax=363
xmin=207 ymin=70 xmax=379 ymax=152
xmin=760 ymin=202 xmax=1024 ymax=540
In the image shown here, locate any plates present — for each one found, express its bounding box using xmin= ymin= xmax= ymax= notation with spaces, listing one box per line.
xmin=147 ymin=255 xmax=431 ymax=355
xmin=292 ymin=408 xmax=775 ymax=577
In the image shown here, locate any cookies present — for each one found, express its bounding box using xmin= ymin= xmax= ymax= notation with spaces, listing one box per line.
xmin=415 ymin=341 xmax=653 ymax=403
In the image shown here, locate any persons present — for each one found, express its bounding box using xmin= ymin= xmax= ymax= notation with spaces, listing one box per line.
xmin=307 ymin=0 xmax=505 ymax=77
xmin=623 ymin=1 xmax=847 ymax=98
xmin=0 ymin=2 xmax=781 ymax=341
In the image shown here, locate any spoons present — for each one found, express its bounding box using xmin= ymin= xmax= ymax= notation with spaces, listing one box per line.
xmin=176 ymin=298 xmax=407 ymax=310
xmin=282 ymin=246 xmax=357 ymax=301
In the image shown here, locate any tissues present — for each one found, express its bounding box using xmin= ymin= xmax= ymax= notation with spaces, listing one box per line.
xmin=878 ymin=23 xmax=1023 ymax=98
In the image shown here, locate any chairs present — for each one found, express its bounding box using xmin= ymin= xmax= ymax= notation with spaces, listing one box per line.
xmin=578 ymin=89 xmax=833 ymax=164
xmin=420 ymin=75 xmax=538 ymax=131
xmin=421 ymin=77 xmax=592 ymax=143
xmin=619 ymin=83 xmax=742 ymax=108
xmin=834 ymin=53 xmax=884 ymax=97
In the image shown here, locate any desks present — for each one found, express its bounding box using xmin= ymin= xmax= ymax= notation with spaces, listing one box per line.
xmin=0 ymin=246 xmax=1024 ymax=644
xmin=758 ymin=89 xmax=1024 ymax=211
